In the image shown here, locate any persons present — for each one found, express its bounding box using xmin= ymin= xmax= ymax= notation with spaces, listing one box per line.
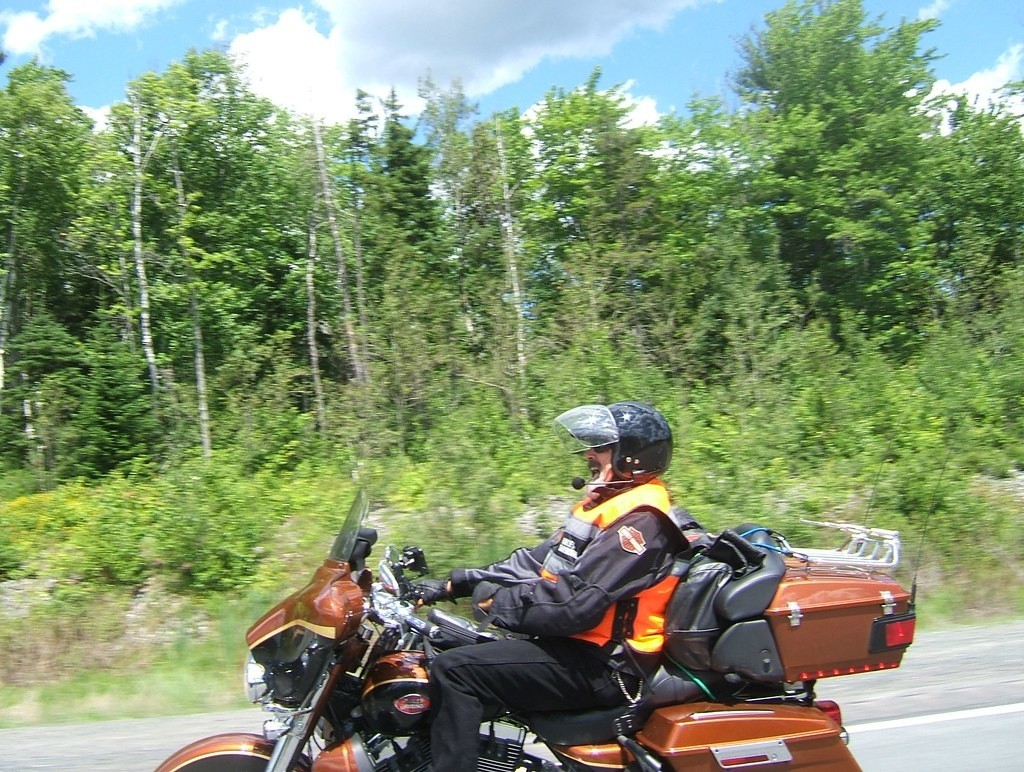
xmin=393 ymin=402 xmax=691 ymax=772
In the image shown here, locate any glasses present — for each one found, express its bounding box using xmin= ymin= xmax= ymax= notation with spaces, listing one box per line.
xmin=593 ymin=438 xmax=613 ymax=453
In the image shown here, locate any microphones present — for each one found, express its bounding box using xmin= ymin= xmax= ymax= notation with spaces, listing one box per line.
xmin=572 ymin=477 xmax=634 ymax=490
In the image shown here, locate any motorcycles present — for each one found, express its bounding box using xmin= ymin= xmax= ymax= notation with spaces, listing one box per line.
xmin=148 ymin=487 xmax=920 ymax=772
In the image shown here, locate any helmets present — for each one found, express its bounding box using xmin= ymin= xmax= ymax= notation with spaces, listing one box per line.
xmin=570 ymin=402 xmax=673 ymax=484
